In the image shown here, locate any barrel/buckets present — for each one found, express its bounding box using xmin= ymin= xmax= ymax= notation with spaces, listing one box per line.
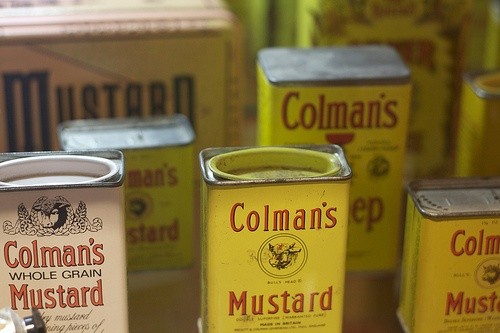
xmin=395 ymin=176 xmax=500 ymax=333
xmin=57 ymin=113 xmax=196 ymax=272
xmin=453 ymin=69 xmax=500 ymax=179
xmin=255 ymin=44 xmax=412 ymax=273
xmin=198 ymin=144 xmax=354 ymax=333
xmin=0 ymin=149 xmax=130 ymax=333
